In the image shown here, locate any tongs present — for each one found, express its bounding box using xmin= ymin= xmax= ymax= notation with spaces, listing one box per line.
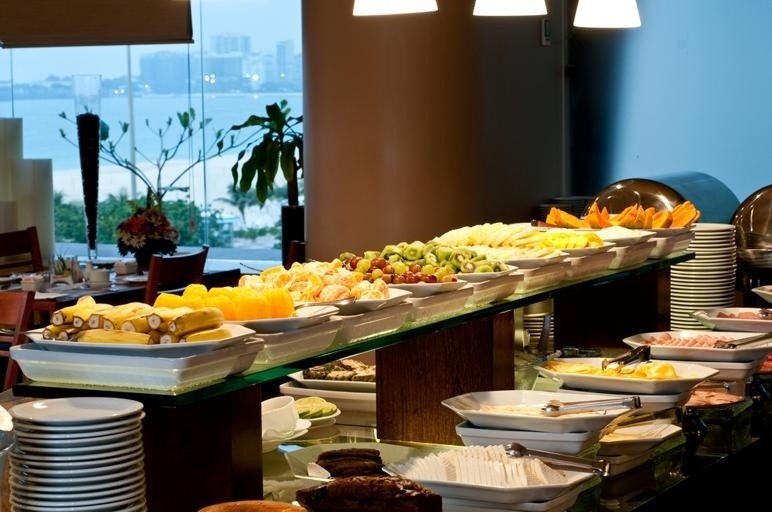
xmin=601 ymin=345 xmax=651 ymax=376
xmin=713 ymin=333 xmax=771 ymax=350
xmin=504 ymin=442 xmax=611 ymax=477
xmin=540 ymin=397 xmax=642 ymax=418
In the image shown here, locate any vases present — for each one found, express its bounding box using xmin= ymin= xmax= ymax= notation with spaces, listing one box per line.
xmin=68 ymin=70 xmax=107 ymax=264
xmin=130 ymin=239 xmax=176 ymax=275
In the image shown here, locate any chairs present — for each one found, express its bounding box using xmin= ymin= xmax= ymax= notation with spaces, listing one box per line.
xmin=0 ymin=287 xmax=36 ymax=397
xmin=0 ymin=224 xmax=47 ymax=278
xmin=25 ymin=300 xmax=57 ymax=329
xmin=143 ymin=243 xmax=211 ymax=306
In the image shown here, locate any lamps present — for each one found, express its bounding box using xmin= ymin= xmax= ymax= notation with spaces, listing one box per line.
xmin=350 ymin=0 xmax=642 ymax=29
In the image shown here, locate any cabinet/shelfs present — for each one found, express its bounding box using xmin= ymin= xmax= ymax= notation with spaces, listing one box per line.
xmin=27 ymin=245 xmax=772 ymax=512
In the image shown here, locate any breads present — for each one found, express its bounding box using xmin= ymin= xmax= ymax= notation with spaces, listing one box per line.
xmin=200 ymin=447 xmax=442 ymax=511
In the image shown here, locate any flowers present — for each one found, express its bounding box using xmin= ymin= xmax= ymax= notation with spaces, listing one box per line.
xmin=115 ymin=206 xmax=181 ymax=252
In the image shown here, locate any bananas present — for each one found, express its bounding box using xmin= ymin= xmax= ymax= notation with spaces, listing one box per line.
xmin=44 ymin=296 xmax=230 ymax=347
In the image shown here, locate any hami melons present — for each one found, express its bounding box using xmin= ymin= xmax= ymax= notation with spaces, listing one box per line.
xmin=530 ymin=201 xmax=701 ymax=228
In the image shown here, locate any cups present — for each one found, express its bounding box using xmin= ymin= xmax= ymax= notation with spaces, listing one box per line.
xmin=15 ymin=252 xmax=147 ymax=294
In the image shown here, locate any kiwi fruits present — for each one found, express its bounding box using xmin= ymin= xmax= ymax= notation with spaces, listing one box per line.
xmin=363 ymin=241 xmax=507 ymax=271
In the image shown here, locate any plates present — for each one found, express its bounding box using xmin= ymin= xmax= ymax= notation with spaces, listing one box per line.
xmin=337 ymin=288 xmax=410 ymax=313
xmin=285 ymin=442 xmax=602 ymax=505
xmin=457 ymin=263 xmax=519 ymax=281
xmin=466 ymin=273 xmax=525 ymax=290
xmin=688 ymin=307 xmax=771 ymax=334
xmin=508 ymin=224 xmax=657 ymax=246
xmin=608 ymin=242 xmax=657 ymax=269
xmin=26 ymin=324 xmax=251 ymax=355
xmin=440 ymin=390 xmax=644 ymax=433
xmin=230 ymin=305 xmax=342 ymax=333
xmin=454 ymin=421 xmax=596 ymax=455
xmin=404 ymin=284 xmax=475 ymax=307
xmin=5 ymin=393 xmax=146 ymax=509
xmin=575 ymin=226 xmax=696 ymax=237
xmin=433 ymin=226 xmax=617 ymax=257
xmin=533 ymin=358 xmax=721 ymax=393
xmin=331 ymin=301 xmax=412 ymax=323
xmin=511 ymin=262 xmax=563 ymax=278
xmin=562 ymin=252 xmax=614 ymax=281
xmin=622 ymin=332 xmax=771 ymax=361
xmin=649 ymin=357 xmax=763 ymax=383
xmin=498 ymin=248 xmax=572 ymax=267
xmin=3 ymin=339 xmax=265 ymax=390
xmin=389 ymin=279 xmax=469 ymax=296
xmin=649 ymin=231 xmax=696 ymax=259
xmin=260 ymin=395 xmax=300 ymax=433
xmin=556 ymin=383 xmax=697 ymax=411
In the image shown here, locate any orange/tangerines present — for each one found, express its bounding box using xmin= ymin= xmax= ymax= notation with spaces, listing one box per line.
xmin=238 ymin=257 xmax=389 ymax=302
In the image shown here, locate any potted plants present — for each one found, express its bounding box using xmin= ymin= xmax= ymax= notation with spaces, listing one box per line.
xmin=216 ymin=96 xmax=305 ymax=264
